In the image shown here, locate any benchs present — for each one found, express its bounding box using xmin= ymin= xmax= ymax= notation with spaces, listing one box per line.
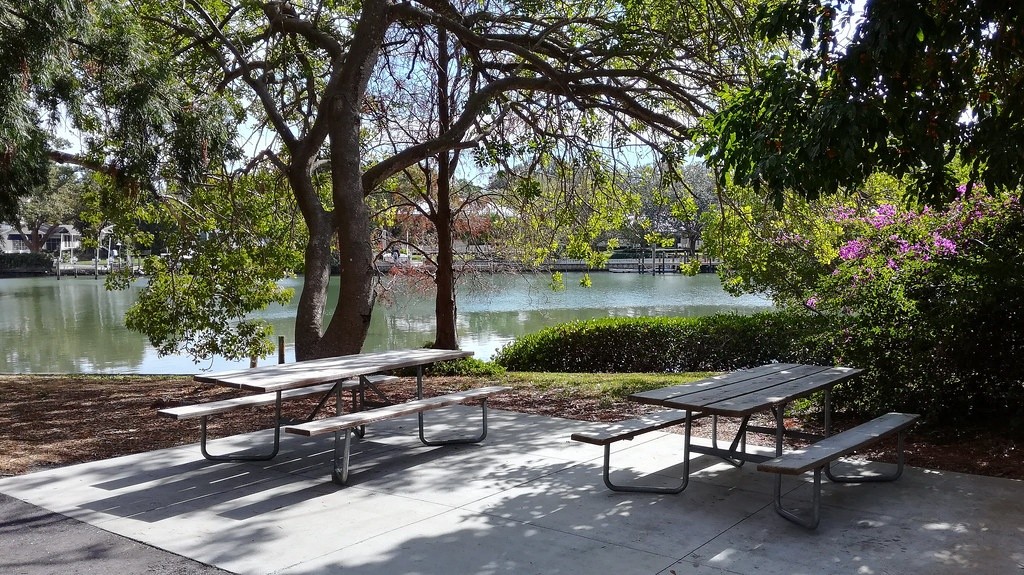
xmin=158 ymin=375 xmax=400 ymax=422
xmin=285 ymin=385 xmax=515 ymax=438
xmin=755 ymin=412 xmax=920 ymax=476
xmin=572 ymin=408 xmax=705 ymax=446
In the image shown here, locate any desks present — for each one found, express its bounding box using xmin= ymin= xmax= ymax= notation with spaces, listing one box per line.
xmin=192 ymin=347 xmax=460 ymax=487
xmin=627 ymin=362 xmax=865 ymax=529
